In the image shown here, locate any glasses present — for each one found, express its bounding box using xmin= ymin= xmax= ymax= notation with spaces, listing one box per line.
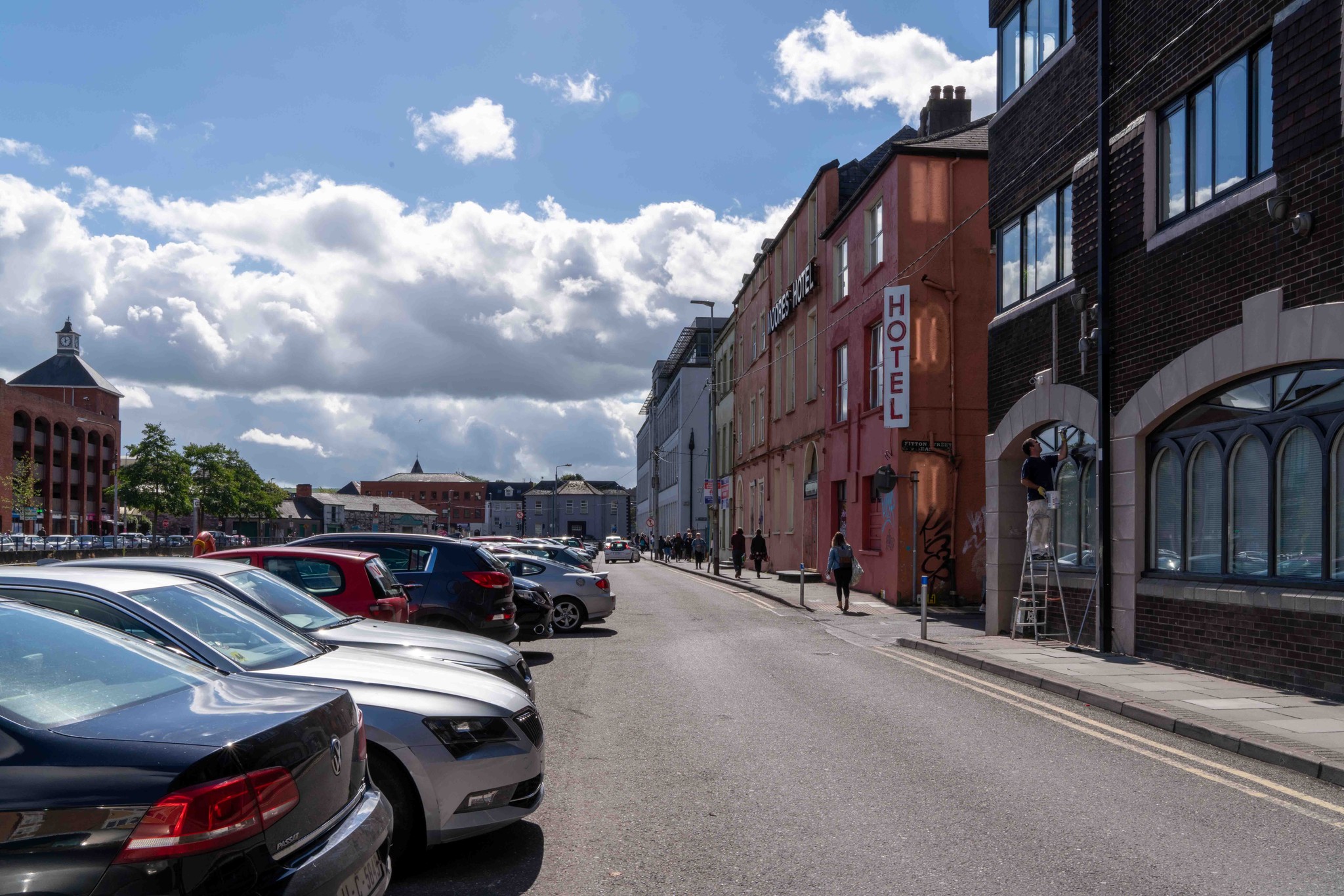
xmin=1031 ymin=440 xmax=1038 ymax=447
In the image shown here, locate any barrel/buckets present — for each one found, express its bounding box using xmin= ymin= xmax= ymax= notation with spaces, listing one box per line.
xmin=1045 ymin=490 xmax=1061 ymax=509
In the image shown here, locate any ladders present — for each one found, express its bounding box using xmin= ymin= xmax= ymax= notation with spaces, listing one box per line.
xmin=1011 ymin=515 xmax=1072 ymax=646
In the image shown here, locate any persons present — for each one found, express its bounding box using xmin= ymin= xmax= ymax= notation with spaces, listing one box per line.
xmin=731 ymin=527 xmax=746 ymax=578
xmin=824 ymin=532 xmax=853 ymax=611
xmin=621 ymin=528 xmax=706 ymax=569
xmin=1021 ymin=427 xmax=1068 ymax=560
xmin=750 ymin=529 xmax=768 ymax=578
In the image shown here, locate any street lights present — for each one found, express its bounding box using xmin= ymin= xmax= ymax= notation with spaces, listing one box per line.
xmin=489 ymin=492 xmax=496 ymax=537
xmin=616 ymin=485 xmax=619 ymax=535
xmin=77 ymin=417 xmax=118 ymax=548
xmin=554 ymin=463 xmax=572 ymax=535
xmin=691 ymin=299 xmax=720 ymax=576
xmin=448 ymin=489 xmax=454 ymax=535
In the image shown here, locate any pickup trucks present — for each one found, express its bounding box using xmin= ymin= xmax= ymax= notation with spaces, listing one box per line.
xmin=203 ymin=531 xmax=235 ymax=546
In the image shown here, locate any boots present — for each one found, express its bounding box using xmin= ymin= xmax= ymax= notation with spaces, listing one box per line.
xmin=844 ymin=597 xmax=849 ymax=611
xmin=837 ymin=601 xmax=842 ymax=608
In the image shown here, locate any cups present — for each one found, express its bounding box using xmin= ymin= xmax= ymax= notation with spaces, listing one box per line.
xmin=826 ymin=574 xmax=831 ymax=579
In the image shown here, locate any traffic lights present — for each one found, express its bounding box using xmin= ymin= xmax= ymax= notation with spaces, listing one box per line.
xmin=431 ymin=523 xmax=434 ymax=530
xmin=516 ymin=524 xmax=519 ymax=531
xmin=499 ymin=523 xmax=502 ymax=529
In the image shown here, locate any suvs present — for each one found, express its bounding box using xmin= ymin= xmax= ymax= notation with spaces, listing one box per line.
xmin=0 ymin=535 xmax=15 ymax=552
xmin=275 ymin=533 xmax=519 ymax=645
xmin=10 ymin=534 xmax=54 ymax=551
xmin=603 ymin=536 xmax=621 ymax=554
xmin=46 ymin=532 xmax=193 ymax=550
xmin=463 ymin=536 xmax=594 ymax=574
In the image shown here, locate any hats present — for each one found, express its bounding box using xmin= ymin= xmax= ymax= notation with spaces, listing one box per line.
xmin=755 ymin=528 xmax=763 ymax=534
xmin=687 ymin=528 xmax=692 ymax=531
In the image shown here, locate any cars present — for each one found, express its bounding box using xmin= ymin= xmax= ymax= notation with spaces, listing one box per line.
xmin=233 ymin=535 xmax=250 ymax=546
xmin=0 ymin=557 xmax=547 ymax=846
xmin=194 ymin=546 xmax=418 ymax=623
xmin=605 ymin=540 xmax=641 ymax=564
xmin=493 ymin=553 xmax=617 ymax=634
xmin=513 ymin=576 xmax=554 ymax=643
xmin=583 ymin=541 xmax=599 ymax=559
xmin=481 ymin=545 xmax=526 ymax=555
xmin=0 ymin=593 xmax=398 ymax=896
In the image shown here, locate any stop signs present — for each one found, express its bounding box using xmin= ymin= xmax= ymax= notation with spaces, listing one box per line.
xmin=162 ymin=520 xmax=169 ymax=527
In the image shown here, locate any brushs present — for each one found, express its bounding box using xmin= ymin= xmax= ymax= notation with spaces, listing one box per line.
xmin=1057 ymin=424 xmax=1072 ymax=433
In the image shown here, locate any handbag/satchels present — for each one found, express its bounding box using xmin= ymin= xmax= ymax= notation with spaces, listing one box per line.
xmin=850 ymin=556 xmax=864 ymax=587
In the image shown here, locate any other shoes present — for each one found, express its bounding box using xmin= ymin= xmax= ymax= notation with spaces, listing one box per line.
xmin=737 ymin=576 xmax=740 ymax=578
xmin=1028 ymin=554 xmax=1045 ymax=560
xmin=659 ymin=556 xmax=692 ymax=564
xmin=695 ymin=565 xmax=698 ymax=568
xmin=1043 ymin=554 xmax=1053 ymax=559
xmin=757 ymin=571 xmax=760 ymax=578
xmin=699 ymin=565 xmax=701 ymax=569
xmin=735 ymin=570 xmax=738 ymax=578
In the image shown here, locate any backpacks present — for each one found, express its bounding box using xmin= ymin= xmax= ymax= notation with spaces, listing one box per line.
xmin=833 ymin=545 xmax=852 ymax=568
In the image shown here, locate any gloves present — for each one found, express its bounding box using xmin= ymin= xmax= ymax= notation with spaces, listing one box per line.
xmin=1059 ymin=427 xmax=1067 ymax=442
xmin=1038 ymin=486 xmax=1047 ymax=499
